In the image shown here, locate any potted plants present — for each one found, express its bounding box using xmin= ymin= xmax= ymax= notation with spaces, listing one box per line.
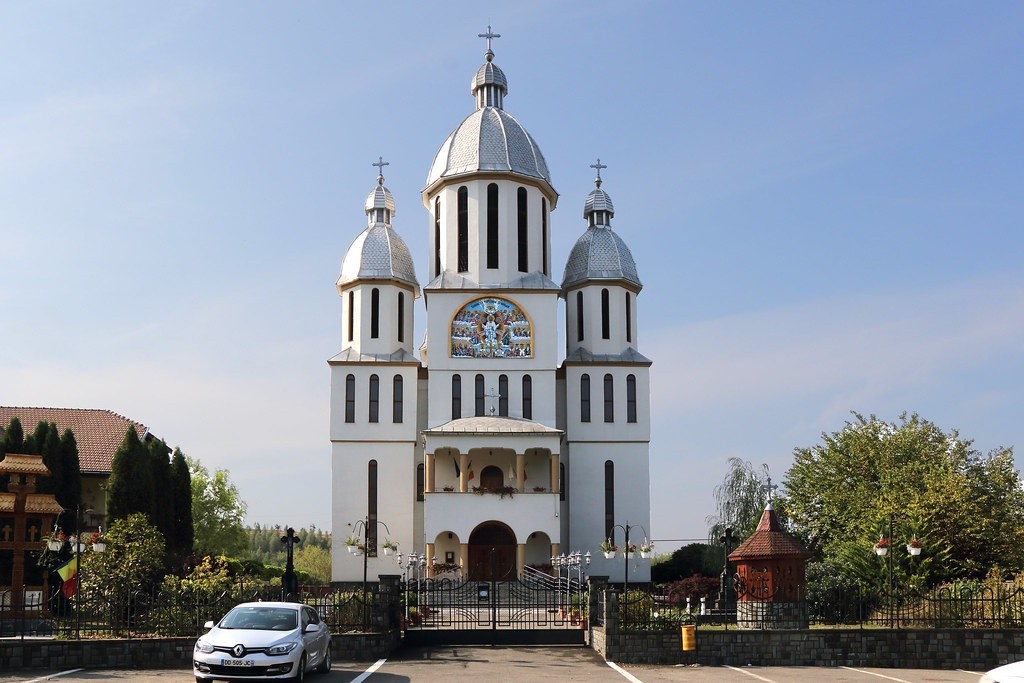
xmin=580 ymin=590 xmax=589 ymax=630
xmin=568 ymin=594 xmax=578 ymax=624
xmin=561 ymin=606 xmax=568 ymax=618
xmin=421 ymin=594 xmax=431 ymax=618
xmin=344 ymin=537 xmax=358 ymax=553
xmin=400 ymin=612 xmax=409 ymax=630
xmin=409 ymin=605 xmax=423 ymax=623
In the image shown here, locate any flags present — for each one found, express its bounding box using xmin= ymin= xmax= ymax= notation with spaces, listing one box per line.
xmin=524 ymin=463 xmax=528 ymax=481
xmin=56 ymin=555 xmax=77 ymax=597
xmin=454 ymin=457 xmax=460 ymax=478
xmin=468 ymin=461 xmax=475 ymax=481
xmin=508 ymin=464 xmax=514 ymax=481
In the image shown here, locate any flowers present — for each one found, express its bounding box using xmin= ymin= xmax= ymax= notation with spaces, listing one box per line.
xmin=906 ymin=540 xmax=925 ymax=551
xmin=598 ymin=540 xmax=617 ymax=553
xmin=640 ymin=541 xmax=657 ymax=552
xmin=355 ymin=543 xmax=365 ymax=549
xmin=873 ymin=537 xmax=890 ymax=553
xmin=42 ymin=527 xmax=109 ymax=543
xmin=380 ymin=541 xmax=400 ymax=551
xmin=621 ymin=545 xmax=637 ymax=553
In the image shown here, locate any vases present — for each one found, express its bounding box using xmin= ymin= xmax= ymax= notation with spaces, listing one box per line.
xmin=354 ymin=548 xmax=362 ymax=556
xmin=72 ymin=543 xmax=87 ymax=553
xmin=93 ymin=543 xmax=108 ymax=553
xmin=876 ymin=547 xmax=887 ymax=556
xmin=603 ymin=550 xmax=615 ymax=559
xmin=911 ymin=547 xmax=921 ymax=556
xmin=48 ymin=542 xmax=61 ymax=552
xmin=641 ymin=550 xmax=656 ymax=559
xmin=384 ymin=548 xmax=394 ymax=557
xmin=623 ymin=551 xmax=634 ymax=559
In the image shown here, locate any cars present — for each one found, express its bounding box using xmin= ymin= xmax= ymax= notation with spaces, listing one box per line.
xmin=192 ymin=599 xmax=332 ymax=683
